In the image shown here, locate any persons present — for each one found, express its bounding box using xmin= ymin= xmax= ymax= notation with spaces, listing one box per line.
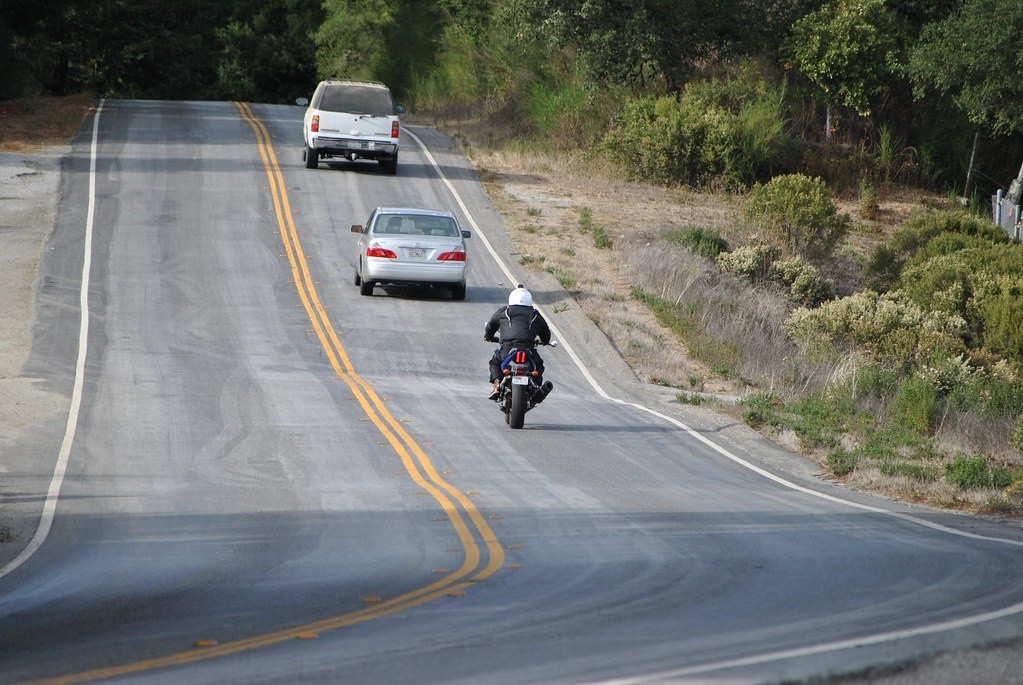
xmin=484 ymin=288 xmax=551 ymax=400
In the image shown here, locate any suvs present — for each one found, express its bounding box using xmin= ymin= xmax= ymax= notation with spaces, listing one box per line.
xmin=295 ymin=79 xmax=407 ymax=173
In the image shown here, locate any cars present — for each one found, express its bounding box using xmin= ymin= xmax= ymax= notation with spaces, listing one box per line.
xmin=350 ymin=206 xmax=471 ymax=300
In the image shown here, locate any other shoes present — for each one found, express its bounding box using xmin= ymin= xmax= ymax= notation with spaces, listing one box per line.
xmin=488 ymin=387 xmax=500 ymax=400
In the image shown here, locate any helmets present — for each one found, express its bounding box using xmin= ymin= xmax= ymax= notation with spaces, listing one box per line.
xmin=508 ymin=288 xmax=533 ymax=306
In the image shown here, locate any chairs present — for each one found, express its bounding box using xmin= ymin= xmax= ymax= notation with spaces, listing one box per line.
xmin=383 ymin=225 xmax=446 ymax=235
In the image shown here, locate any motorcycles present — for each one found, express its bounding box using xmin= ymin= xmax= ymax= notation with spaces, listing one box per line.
xmin=484 ymin=322 xmax=558 ymax=428
